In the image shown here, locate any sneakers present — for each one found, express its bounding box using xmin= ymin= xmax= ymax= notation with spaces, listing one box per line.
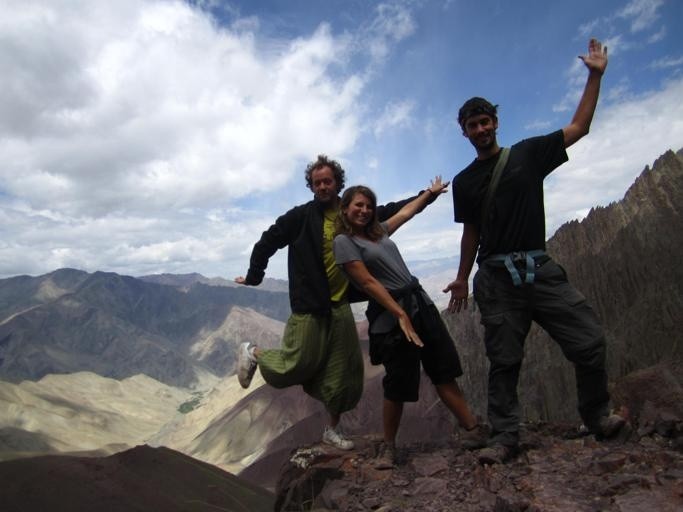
xmin=322 ymin=428 xmax=354 ymax=450
xmin=581 ymin=414 xmax=625 ymax=439
xmin=237 ymin=342 xmax=259 ymax=388
xmin=473 ymin=441 xmax=514 ymax=463
xmin=376 ymin=442 xmax=396 ymax=469
xmin=456 ymin=423 xmax=488 ymax=440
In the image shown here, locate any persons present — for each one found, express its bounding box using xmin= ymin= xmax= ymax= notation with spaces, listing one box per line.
xmin=443 ymin=38 xmax=628 ymax=466
xmin=331 ymin=174 xmax=478 ymax=470
xmin=235 ymin=153 xmax=450 ymax=450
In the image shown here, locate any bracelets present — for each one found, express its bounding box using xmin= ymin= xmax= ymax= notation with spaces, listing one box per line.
xmin=427 ymin=188 xmax=434 ymax=197
